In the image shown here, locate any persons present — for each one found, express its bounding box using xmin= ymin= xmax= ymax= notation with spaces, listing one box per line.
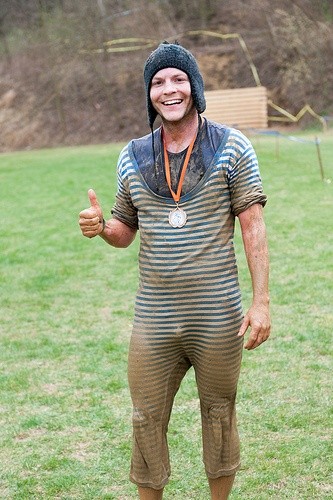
xmin=80 ymin=40 xmax=271 ymax=500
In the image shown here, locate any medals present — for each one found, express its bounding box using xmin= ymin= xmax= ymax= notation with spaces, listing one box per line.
xmin=168 ymin=208 xmax=187 ymax=228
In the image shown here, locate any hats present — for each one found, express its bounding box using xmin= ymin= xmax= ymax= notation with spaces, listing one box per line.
xmin=144 ymin=39 xmax=207 ymax=129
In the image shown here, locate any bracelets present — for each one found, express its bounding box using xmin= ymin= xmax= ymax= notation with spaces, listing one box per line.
xmin=103 ymin=219 xmax=105 ymax=232
